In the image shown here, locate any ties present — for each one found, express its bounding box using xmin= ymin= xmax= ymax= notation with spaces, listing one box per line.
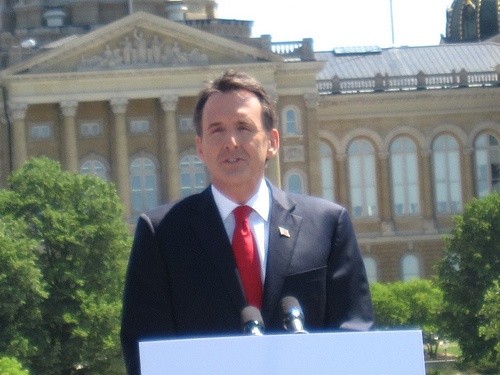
xmin=231 ymin=205 xmax=264 ymax=312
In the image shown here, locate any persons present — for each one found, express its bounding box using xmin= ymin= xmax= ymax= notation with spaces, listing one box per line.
xmin=120 ymin=68 xmax=374 ymax=375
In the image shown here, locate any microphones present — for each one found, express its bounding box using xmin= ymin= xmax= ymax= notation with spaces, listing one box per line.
xmin=240 ymin=306 xmax=265 ymax=335
xmin=278 ymin=296 xmax=309 ymax=334
